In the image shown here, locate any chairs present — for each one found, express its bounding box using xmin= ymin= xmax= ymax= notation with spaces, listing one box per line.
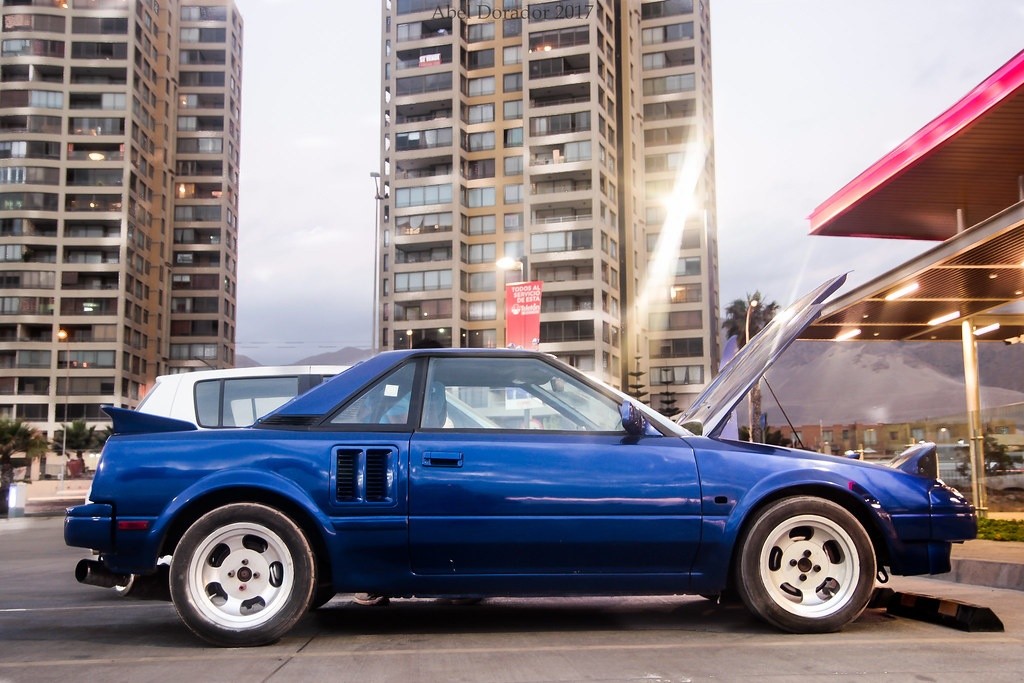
xmin=426 ymin=380 xmax=448 ymax=427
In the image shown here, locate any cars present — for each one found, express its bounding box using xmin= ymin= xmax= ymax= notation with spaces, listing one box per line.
xmin=62 ymin=268 xmax=979 ymax=650
xmin=132 ymin=364 xmax=502 ymax=428
